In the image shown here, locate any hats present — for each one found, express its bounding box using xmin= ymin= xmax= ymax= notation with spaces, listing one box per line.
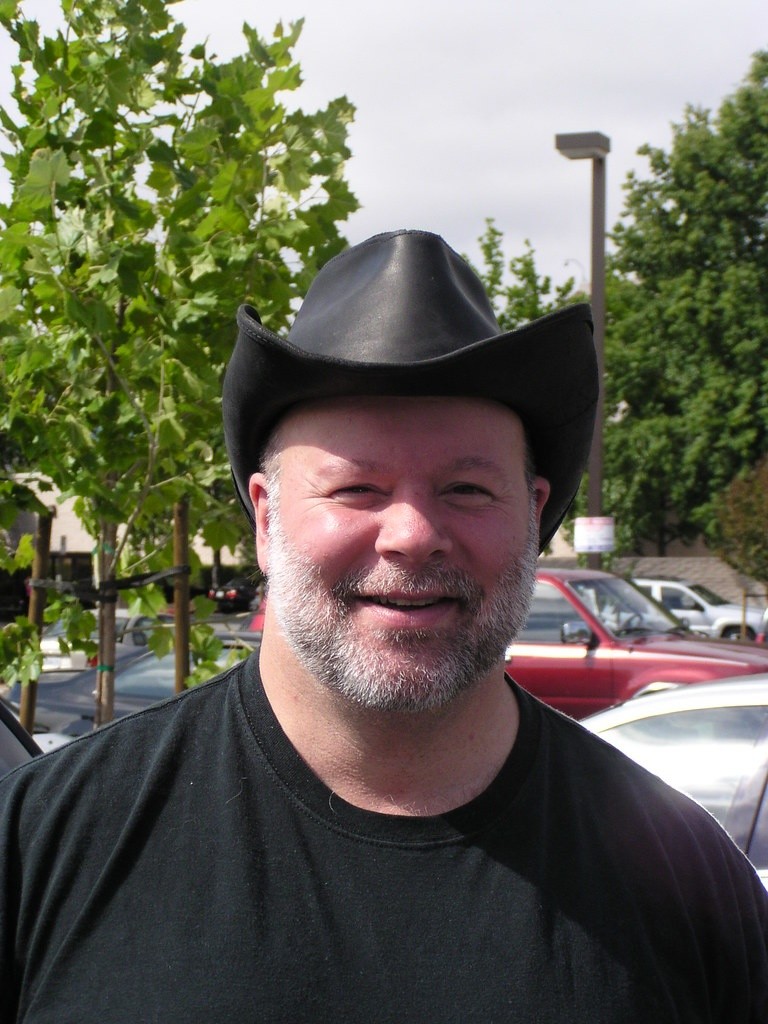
xmin=218 ymin=229 xmax=603 ymax=554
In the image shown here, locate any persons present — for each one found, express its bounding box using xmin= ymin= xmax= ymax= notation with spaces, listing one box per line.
xmin=0 ymin=230 xmax=768 ymax=1024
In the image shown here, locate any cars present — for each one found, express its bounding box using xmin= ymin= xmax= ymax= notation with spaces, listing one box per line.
xmin=532 ymin=574 xmax=768 ymax=644
xmin=573 ymin=670 xmax=768 ymax=897
xmin=0 ymin=566 xmax=266 ymax=787
xmin=231 ymin=569 xmax=768 ymax=721
xmin=207 ymin=568 xmax=266 ymax=613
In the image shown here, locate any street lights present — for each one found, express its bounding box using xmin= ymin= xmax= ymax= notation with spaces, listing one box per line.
xmin=555 ymin=127 xmax=611 ymax=564
